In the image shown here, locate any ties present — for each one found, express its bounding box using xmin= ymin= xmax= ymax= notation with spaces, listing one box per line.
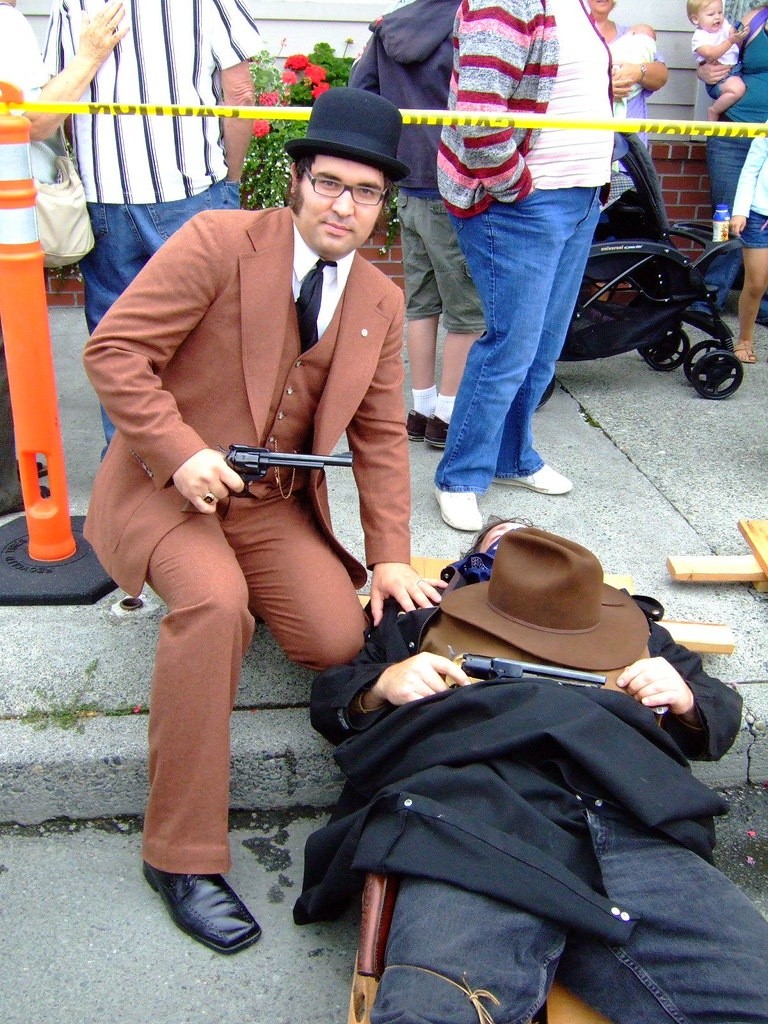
xmin=294 ymin=259 xmax=337 ymax=354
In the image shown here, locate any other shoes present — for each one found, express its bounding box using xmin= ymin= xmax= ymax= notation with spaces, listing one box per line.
xmin=425 ymin=413 xmax=450 ymax=450
xmin=404 ymin=408 xmax=429 ymax=444
xmin=488 ymin=461 xmax=574 ymax=496
xmin=434 ymin=486 xmax=483 ymax=533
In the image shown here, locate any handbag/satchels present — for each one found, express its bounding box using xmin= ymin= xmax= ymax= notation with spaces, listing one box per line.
xmin=32 ymin=155 xmax=94 ymax=269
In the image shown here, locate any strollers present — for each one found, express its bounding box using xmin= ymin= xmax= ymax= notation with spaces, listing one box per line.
xmin=534 ymin=129 xmax=746 ymax=413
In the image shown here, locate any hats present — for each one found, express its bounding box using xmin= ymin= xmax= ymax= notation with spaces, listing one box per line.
xmin=440 ymin=527 xmax=652 ymax=670
xmin=283 ymin=85 xmax=410 ymax=186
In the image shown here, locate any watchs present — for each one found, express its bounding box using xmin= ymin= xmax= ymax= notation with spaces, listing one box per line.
xmin=638 ymin=63 xmax=646 ymax=83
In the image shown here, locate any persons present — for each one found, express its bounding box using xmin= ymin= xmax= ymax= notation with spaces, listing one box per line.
xmin=697 ymin=0 xmax=768 ymax=363
xmin=41 ymin=0 xmax=266 ymax=462
xmin=293 ymin=515 xmax=768 ymax=1024
xmin=434 ymin=0 xmax=615 ymax=531
xmin=86 ymin=88 xmax=451 ymax=955
xmin=0 ymin=0 xmax=130 ymax=517
xmin=587 ymin=0 xmax=668 ymax=188
xmin=348 ymin=0 xmax=487 ymax=449
xmin=687 ymin=0 xmax=750 ymax=121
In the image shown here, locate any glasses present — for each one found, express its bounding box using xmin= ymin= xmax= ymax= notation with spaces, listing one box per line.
xmin=303 ymin=164 xmax=390 ymax=206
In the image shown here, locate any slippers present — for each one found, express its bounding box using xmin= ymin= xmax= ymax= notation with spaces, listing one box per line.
xmin=733 ymin=341 xmax=758 ymax=364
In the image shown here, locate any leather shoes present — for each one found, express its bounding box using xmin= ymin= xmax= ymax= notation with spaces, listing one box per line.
xmin=143 ymin=858 xmax=261 ymax=954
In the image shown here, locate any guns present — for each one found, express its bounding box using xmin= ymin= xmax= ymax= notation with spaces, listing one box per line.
xmin=224 ymin=442 xmax=355 ymax=484
xmin=442 ymin=645 xmax=608 ymax=692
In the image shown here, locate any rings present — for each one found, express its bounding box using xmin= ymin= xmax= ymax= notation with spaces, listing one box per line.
xmin=109 ymin=25 xmax=115 ymax=28
xmin=204 ymin=493 xmax=214 ymax=503
xmin=417 ymin=580 xmax=425 ymax=584
